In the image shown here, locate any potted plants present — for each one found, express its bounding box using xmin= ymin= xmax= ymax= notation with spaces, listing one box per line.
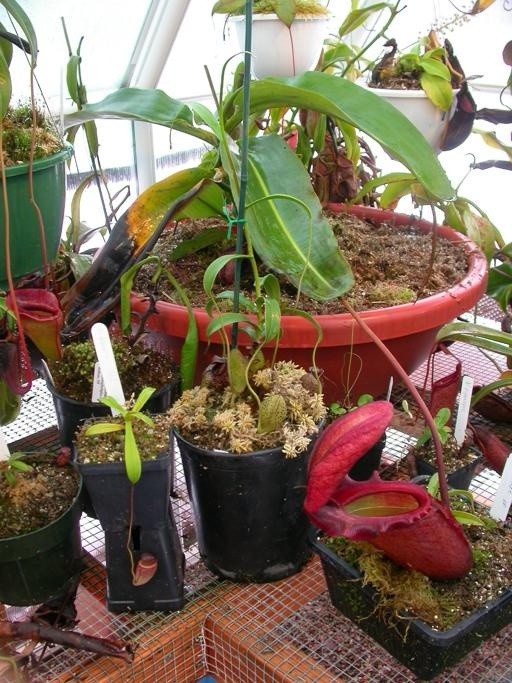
xmin=289 ymin=390 xmax=512 ymax=682
xmin=405 ymin=390 xmax=484 ymax=494
xmin=206 ymin=0 xmax=328 ymax=88
xmin=54 ymin=26 xmax=489 ymax=413
xmin=350 ymin=15 xmax=471 ymax=162
xmin=153 ymin=1 xmax=333 ymax=588
xmin=0 ymin=368 xmax=86 ymax=612
xmin=0 ymin=3 xmax=87 ymax=286
xmin=61 ymin=383 xmax=186 ymax=618
